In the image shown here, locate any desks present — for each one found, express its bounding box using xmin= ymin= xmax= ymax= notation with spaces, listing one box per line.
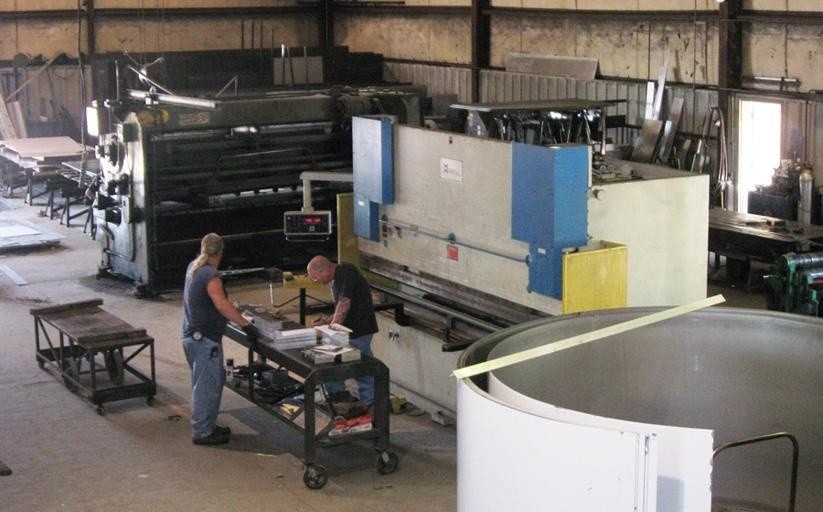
xmin=31 ymin=292 xmax=156 ymax=412
xmin=219 ymin=302 xmax=401 ymax=493
xmin=709 ymin=207 xmax=823 ymax=288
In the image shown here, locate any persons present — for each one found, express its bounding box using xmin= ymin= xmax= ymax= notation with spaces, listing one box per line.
xmin=306 ymin=255 xmax=378 ymax=408
xmin=180 ymin=232 xmax=260 ymax=445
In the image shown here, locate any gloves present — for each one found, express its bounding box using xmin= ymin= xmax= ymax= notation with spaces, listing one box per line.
xmin=242 ymin=324 xmax=261 ymax=342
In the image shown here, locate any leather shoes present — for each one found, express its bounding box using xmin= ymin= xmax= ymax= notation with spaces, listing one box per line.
xmin=193 ymin=434 xmax=230 ymax=445
xmin=212 ymin=425 xmax=231 ymax=435
